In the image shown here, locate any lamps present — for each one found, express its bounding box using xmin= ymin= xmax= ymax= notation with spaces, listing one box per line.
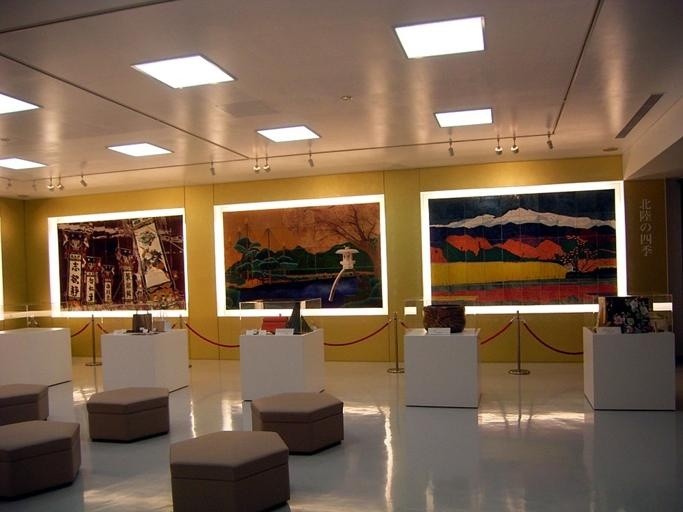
xmin=447 ymin=133 xmax=553 ymax=156
xmin=211 ymin=153 xmax=313 ymax=175
xmin=7 ymin=175 xmax=87 ymax=192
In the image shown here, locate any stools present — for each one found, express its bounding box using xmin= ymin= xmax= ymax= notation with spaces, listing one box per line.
xmin=88 ymin=387 xmax=170 ymax=443
xmin=251 ymin=392 xmax=343 ymax=454
xmin=170 ymin=430 xmax=289 ymax=511
xmin=1 ymin=420 xmax=81 ymax=501
xmin=2 ymin=383 xmax=48 ymax=426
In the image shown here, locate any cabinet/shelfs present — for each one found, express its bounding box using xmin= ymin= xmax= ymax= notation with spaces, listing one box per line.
xmin=404 ymin=327 xmax=479 ymax=406
xmin=102 ymin=327 xmax=193 ymax=393
xmin=582 ymin=292 xmax=677 ymax=412
xmin=239 ymin=329 xmax=324 ymax=398
xmin=3 ymin=328 xmax=75 ymax=395
